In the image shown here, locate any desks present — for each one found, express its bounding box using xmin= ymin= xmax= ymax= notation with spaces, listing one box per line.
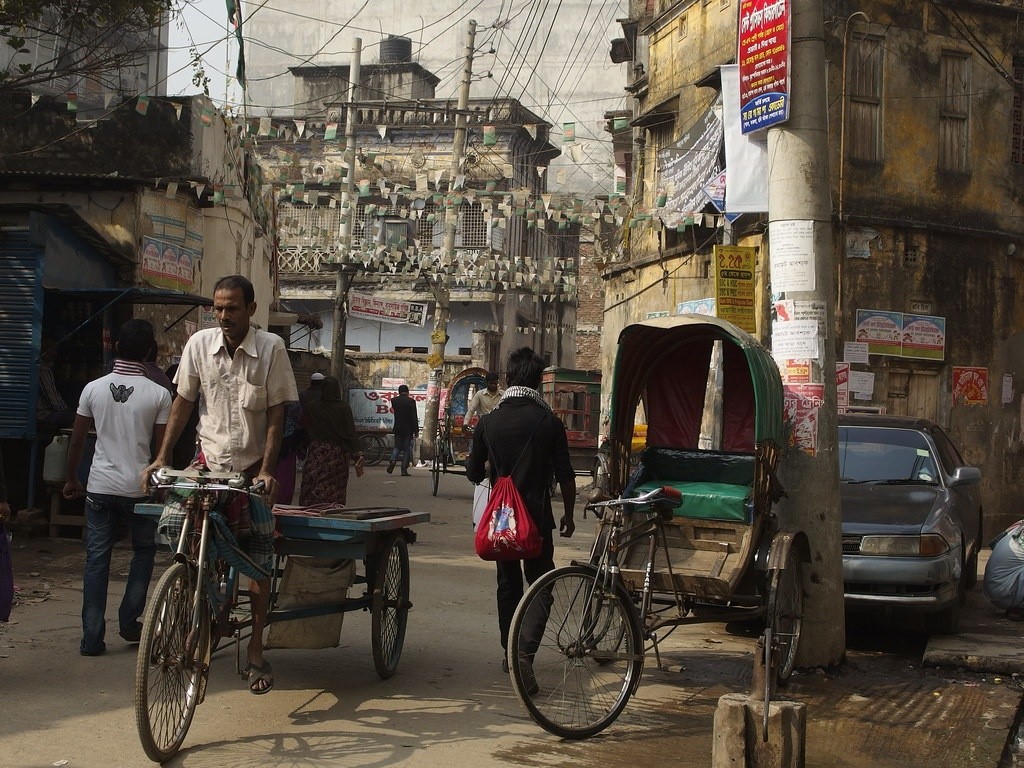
xmin=49 ymin=429 xmax=97 ymax=541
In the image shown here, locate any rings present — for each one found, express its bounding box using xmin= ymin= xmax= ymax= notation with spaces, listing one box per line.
xmin=0 ymin=514 xmax=3 ymax=517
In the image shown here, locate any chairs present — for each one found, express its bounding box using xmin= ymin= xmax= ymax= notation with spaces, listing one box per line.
xmin=874 ymin=448 xmax=936 ymax=479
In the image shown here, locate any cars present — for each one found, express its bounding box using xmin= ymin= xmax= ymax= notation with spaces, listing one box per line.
xmin=730 ymin=411 xmax=985 ymax=653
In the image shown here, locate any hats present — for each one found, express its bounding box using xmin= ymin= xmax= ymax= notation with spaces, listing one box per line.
xmin=311 ymin=372 xmax=327 ymax=380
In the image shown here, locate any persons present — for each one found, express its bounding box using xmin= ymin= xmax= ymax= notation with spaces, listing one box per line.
xmin=63 ymin=320 xmax=172 ymax=655
xmin=146 ymin=340 xmax=173 ymax=393
xmin=467 ymin=347 xmax=576 ymax=694
xmin=462 ymin=372 xmax=506 ymax=433
xmin=386 ymin=385 xmax=419 ymax=476
xmin=983 ymin=519 xmax=1024 ymax=620
xmin=165 ymin=364 xmax=199 ymax=471
xmin=143 ymin=275 xmax=298 ymax=694
xmin=40 ymin=339 xmax=74 ymax=425
xmin=0 ymin=442 xmax=13 ymax=623
xmin=274 ymin=372 xmax=356 ymax=506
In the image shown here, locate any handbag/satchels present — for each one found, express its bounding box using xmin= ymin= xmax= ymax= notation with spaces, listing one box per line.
xmin=265 ymin=557 xmax=355 ymax=649
xmin=0 ymin=520 xmax=14 ymax=622
xmin=355 ymin=458 xmax=364 ymax=477
xmin=471 ymin=478 xmax=492 ymax=532
xmin=409 ymin=436 xmax=420 ymax=467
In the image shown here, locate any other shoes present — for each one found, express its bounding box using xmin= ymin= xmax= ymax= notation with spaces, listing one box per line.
xmin=1008 ymin=608 xmax=1024 ymax=621
xmin=119 ymin=622 xmax=142 ymax=641
xmin=502 ymin=659 xmax=509 ymax=672
xmin=81 ymin=642 xmax=106 ymax=656
xmin=517 ymin=651 xmax=539 ymax=694
xmin=401 ymin=473 xmax=412 ymax=476
xmin=387 ymin=461 xmax=396 ymax=474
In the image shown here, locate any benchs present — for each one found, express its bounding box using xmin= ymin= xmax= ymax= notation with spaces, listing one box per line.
xmin=450 ymin=415 xmax=479 ymax=439
xmin=632 ymin=447 xmax=756 ymax=522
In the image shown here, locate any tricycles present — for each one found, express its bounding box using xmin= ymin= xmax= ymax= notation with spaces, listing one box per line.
xmin=429 ymin=365 xmax=497 ymax=499
xmin=502 ymin=309 xmax=815 ymax=743
xmin=129 ymin=467 xmax=429 ymax=763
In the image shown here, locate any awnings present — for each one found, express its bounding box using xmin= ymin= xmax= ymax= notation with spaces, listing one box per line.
xmin=36 ymin=286 xmax=215 ymax=363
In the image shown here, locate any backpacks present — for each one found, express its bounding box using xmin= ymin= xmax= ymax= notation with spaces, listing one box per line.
xmin=474 ymin=410 xmax=544 ymax=561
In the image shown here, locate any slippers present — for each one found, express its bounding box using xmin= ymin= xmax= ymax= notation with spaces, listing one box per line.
xmin=248 ymin=660 xmax=274 ymax=694
